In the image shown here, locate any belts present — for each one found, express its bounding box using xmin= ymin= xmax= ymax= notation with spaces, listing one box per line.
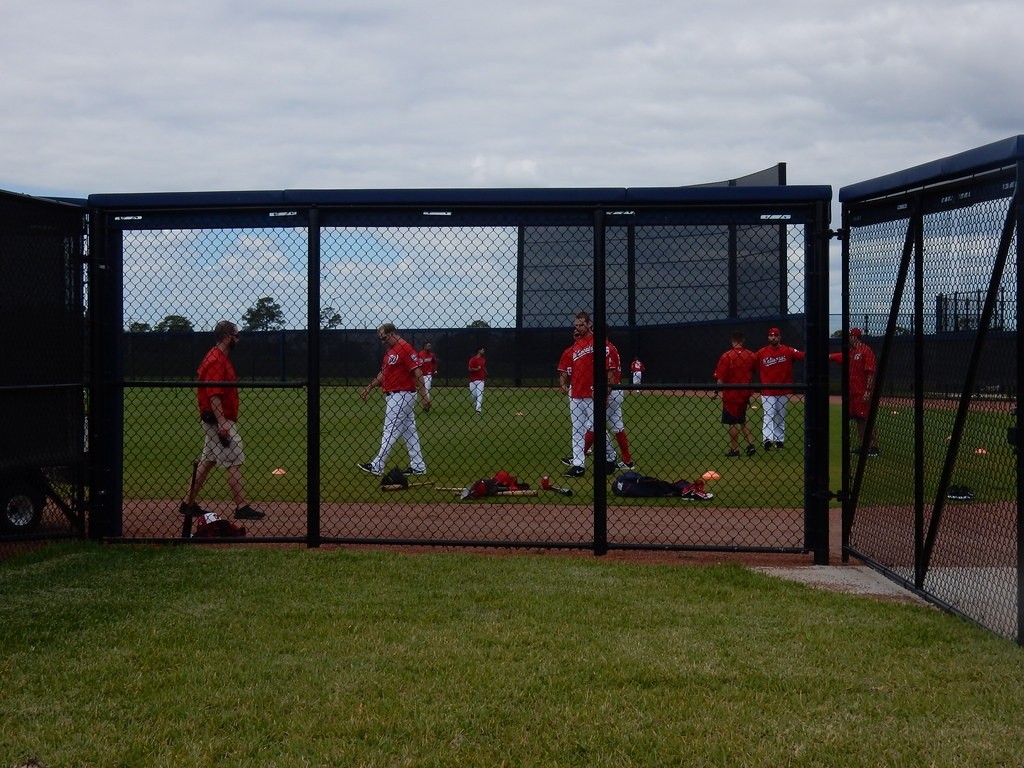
xmin=385 ymin=389 xmax=416 ymax=395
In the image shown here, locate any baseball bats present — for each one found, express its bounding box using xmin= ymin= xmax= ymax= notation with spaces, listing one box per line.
xmin=381 ymin=481 xmax=435 ymax=491
xmin=436 ymin=486 xmax=511 ymax=492
xmin=455 ymin=490 xmax=538 ymax=496
xmin=182 ymin=461 xmax=199 ymax=537
xmin=549 ymin=485 xmax=572 ymax=496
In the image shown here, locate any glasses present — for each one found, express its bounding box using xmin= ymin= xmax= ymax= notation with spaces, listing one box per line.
xmin=380 ymin=334 xmax=389 ymax=341
xmin=229 ymin=333 xmax=241 ymax=339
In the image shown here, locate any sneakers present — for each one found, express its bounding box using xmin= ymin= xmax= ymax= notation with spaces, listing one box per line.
xmin=615 ymin=460 xmax=636 ymax=471
xmin=866 ymin=447 xmax=879 ymax=457
xmin=358 ymin=462 xmax=382 ymax=476
xmin=606 ymin=454 xmax=621 ymax=475
xmin=178 ymin=500 xmax=212 ymax=517
xmin=744 ymin=444 xmax=757 ymax=456
xmin=562 ymin=466 xmax=587 ymax=478
xmin=403 ymin=466 xmax=427 ymax=475
xmin=560 ymin=456 xmax=577 ymax=466
xmin=233 ymin=505 xmax=267 ymax=519
xmin=725 ymin=448 xmax=744 ymax=459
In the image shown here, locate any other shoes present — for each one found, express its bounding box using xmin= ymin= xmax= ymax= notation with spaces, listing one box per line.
xmin=764 ymin=441 xmax=777 ymax=451
xmin=774 ymin=442 xmax=784 ymax=450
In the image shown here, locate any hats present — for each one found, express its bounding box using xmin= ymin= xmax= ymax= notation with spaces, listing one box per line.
xmin=767 ymin=327 xmax=781 ymax=336
xmin=849 ymin=328 xmax=862 ymax=337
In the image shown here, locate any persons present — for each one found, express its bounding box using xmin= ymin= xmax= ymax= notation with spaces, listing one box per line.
xmin=418 ymin=341 xmax=437 ymax=412
xmin=631 ymin=357 xmax=645 ymax=394
xmin=830 ymin=327 xmax=880 ymax=456
xmin=755 ymin=327 xmax=805 ymax=449
xmin=556 ymin=311 xmax=635 ymax=478
xmin=711 ymin=331 xmax=757 ymax=456
xmin=467 ymin=347 xmax=488 ymax=414
xmin=355 ymin=324 xmax=431 ymax=477
xmin=178 ymin=321 xmax=267 ymax=521
